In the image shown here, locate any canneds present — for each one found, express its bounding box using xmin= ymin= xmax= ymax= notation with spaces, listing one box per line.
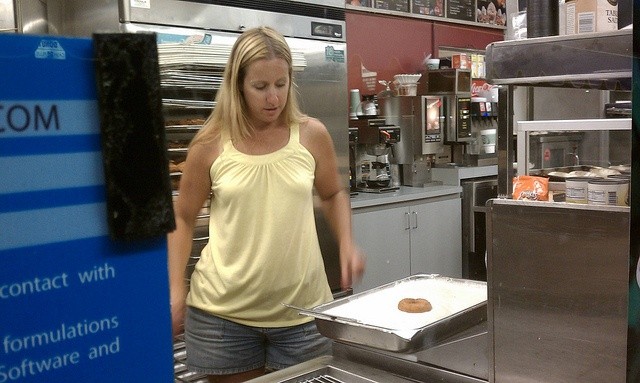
xmin=565 ymin=177 xmax=603 ymax=204
xmin=587 ymin=178 xmax=630 ymax=205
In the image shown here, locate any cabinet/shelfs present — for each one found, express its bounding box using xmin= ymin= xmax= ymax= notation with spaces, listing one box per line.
xmin=345 ymin=0 xmax=507 ymax=29
xmin=343 ymin=11 xmax=506 ymax=128
xmin=516 ymin=118 xmax=632 ymax=179
xmin=432 ymin=164 xmax=498 ymax=186
xmin=350 ymin=182 xmax=463 ymax=295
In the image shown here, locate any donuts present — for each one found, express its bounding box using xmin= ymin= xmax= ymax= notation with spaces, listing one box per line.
xmin=398 ymin=298 xmax=432 ymax=313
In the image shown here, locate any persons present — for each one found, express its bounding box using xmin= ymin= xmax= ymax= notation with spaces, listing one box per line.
xmin=161 ymin=26 xmax=366 ymax=382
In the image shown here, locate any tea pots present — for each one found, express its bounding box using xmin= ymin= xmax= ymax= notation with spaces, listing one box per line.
xmin=357 ymin=95 xmax=379 ymax=120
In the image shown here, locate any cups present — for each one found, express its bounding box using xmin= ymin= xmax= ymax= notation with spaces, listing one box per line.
xmin=480 ymin=128 xmax=497 ymax=154
xmin=349 ymin=88 xmax=365 ymax=118
xmin=425 ymin=58 xmax=440 ymax=71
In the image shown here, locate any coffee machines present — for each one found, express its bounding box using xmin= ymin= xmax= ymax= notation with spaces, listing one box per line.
xmin=350 ymin=115 xmax=401 ymax=196
xmin=377 ymin=93 xmax=446 ymax=188
xmin=416 ymin=69 xmax=475 ymax=187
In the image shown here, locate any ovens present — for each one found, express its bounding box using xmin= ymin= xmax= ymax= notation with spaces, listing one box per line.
xmin=46 ymin=2 xmax=353 ymax=383
